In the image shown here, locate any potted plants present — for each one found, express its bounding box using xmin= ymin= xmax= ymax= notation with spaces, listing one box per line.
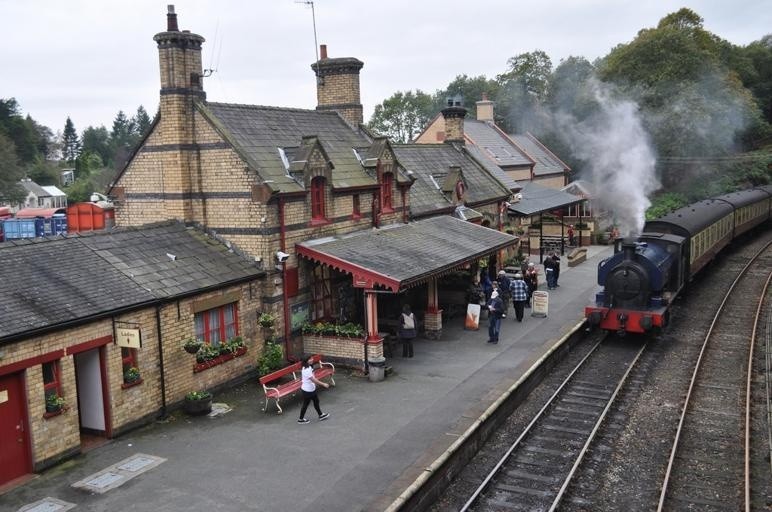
xmin=124 ymin=367 xmax=140 ymax=383
xmin=184 ymin=336 xmax=201 ymax=354
xmin=183 ymin=392 xmax=212 ymax=413
xmin=46 ymin=394 xmax=64 ymax=414
xmin=258 ymin=313 xmax=274 ymax=328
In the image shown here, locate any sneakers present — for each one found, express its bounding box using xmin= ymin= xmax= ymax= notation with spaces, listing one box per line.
xmin=298 ymin=418 xmax=310 ymax=423
xmin=319 ymin=413 xmax=329 ymax=420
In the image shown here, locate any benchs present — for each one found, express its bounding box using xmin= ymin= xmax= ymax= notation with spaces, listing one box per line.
xmin=259 ymin=354 xmax=336 ymax=415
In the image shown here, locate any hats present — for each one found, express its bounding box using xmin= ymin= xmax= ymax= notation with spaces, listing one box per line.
xmin=491 ymin=291 xmax=499 ymax=299
xmin=499 ymin=270 xmax=505 ymax=274
xmin=512 ymin=274 xmax=520 ymax=278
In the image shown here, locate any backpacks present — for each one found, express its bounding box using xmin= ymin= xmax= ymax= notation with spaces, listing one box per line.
xmin=402 ymin=313 xmax=414 ymax=330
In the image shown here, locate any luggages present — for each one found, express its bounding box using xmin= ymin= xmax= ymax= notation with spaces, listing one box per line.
xmin=532 ymin=291 xmax=548 ymax=317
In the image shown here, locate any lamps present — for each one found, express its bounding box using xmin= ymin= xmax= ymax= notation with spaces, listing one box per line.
xmin=276 ymin=252 xmax=290 ymax=262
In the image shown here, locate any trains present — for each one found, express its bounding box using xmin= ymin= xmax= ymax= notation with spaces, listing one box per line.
xmin=584 ymin=181 xmax=772 ymax=347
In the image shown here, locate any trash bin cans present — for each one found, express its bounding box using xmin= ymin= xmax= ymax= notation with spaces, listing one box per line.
xmin=367 ymin=356 xmax=386 ymax=383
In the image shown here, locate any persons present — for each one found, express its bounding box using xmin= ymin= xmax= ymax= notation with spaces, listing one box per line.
xmin=467 ymin=250 xmax=561 ymax=343
xmin=296 ymin=353 xmax=331 ymax=424
xmin=399 ymin=304 xmax=417 ymax=358
xmin=567 ymin=223 xmax=575 ymax=246
xmin=610 ymin=226 xmax=620 ymax=239
